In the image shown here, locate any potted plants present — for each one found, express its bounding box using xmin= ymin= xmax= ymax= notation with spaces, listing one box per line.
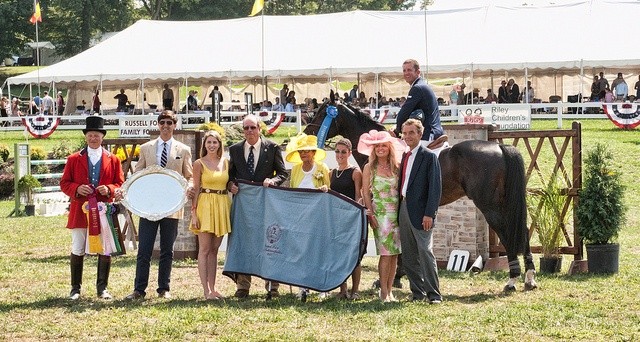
xmin=527 ymin=164 xmax=576 ymax=275
xmin=570 ymin=141 xmax=628 ymax=275
xmin=18 ymin=175 xmax=42 ymax=217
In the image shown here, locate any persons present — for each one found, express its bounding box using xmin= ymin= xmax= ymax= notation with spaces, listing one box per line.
xmin=113 ymin=88 xmax=131 ymax=112
xmin=288 ymin=135 xmax=331 ymax=301
xmin=93 ymin=88 xmax=102 ymax=113
xmin=361 ymin=130 xmax=402 ymax=303
xmin=189 ymin=130 xmax=232 ymax=301
xmin=209 ymin=86 xmax=223 ymax=109
xmin=0 ymin=90 xmax=65 ymax=117
xmin=226 ymin=113 xmax=290 ymax=300
xmin=113 ymin=109 xmax=195 ymax=301
xmin=229 ymin=83 xmax=406 ymax=125
xmin=397 ymin=118 xmax=443 ymax=304
xmin=186 ymin=90 xmax=198 ymax=109
xmin=590 ymin=71 xmax=640 ymax=100
xmin=447 ymin=78 xmax=535 ymax=104
xmin=59 ymin=116 xmax=124 ymax=300
xmin=329 ymin=138 xmax=372 ymax=301
xmin=395 ymin=58 xmax=444 ymax=143
xmin=161 ymin=84 xmax=174 ymax=110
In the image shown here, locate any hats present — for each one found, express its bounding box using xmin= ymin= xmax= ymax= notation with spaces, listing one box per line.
xmin=82 ymin=116 xmax=106 ymax=136
xmin=360 ymin=130 xmax=398 ymax=148
xmin=285 ymin=135 xmax=327 ymax=164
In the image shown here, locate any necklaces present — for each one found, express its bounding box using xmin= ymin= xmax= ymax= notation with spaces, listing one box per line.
xmin=378 ymin=162 xmax=391 ymax=170
xmin=303 ymin=164 xmax=313 ymax=171
xmin=335 ymin=164 xmax=350 ymax=178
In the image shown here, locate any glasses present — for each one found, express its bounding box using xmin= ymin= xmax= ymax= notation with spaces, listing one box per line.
xmin=158 ymin=121 xmax=174 ymax=126
xmin=87 ymin=134 xmax=99 ymax=138
xmin=298 ymin=150 xmax=310 ymax=153
xmin=335 ymin=150 xmax=348 ymax=154
xmin=243 ymin=125 xmax=257 ymax=130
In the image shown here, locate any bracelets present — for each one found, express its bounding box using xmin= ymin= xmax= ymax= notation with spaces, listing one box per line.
xmin=191 ymin=207 xmax=197 ymax=209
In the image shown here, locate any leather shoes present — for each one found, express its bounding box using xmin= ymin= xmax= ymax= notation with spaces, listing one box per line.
xmin=405 ymin=296 xmax=421 ymax=302
xmin=123 ymin=290 xmax=144 ymax=301
xmin=235 ymin=289 xmax=250 ymax=298
xmin=267 ymin=290 xmax=280 ymax=299
xmin=429 ymin=298 xmax=441 ymax=304
xmin=158 ymin=290 xmax=172 ymax=300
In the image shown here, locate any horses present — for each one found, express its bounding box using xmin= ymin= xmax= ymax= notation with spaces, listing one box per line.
xmin=302 ymin=90 xmax=538 ymax=295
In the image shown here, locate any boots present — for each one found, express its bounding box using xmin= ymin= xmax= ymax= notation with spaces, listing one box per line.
xmin=97 ymin=254 xmax=112 ymax=299
xmin=67 ymin=252 xmax=84 ymax=300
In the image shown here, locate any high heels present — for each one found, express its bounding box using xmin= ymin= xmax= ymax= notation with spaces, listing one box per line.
xmin=378 ymin=289 xmax=390 ymax=304
xmin=388 ymin=291 xmax=400 ymax=302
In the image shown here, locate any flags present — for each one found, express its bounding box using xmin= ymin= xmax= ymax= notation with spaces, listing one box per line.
xmin=247 ymin=0 xmax=264 ymax=17
xmin=29 ymin=2 xmax=42 ymax=25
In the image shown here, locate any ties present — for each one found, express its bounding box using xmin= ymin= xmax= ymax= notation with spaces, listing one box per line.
xmin=247 ymin=146 xmax=255 ymax=178
xmin=400 ymin=151 xmax=412 ymax=198
xmin=160 ymin=143 xmax=168 ymax=168
